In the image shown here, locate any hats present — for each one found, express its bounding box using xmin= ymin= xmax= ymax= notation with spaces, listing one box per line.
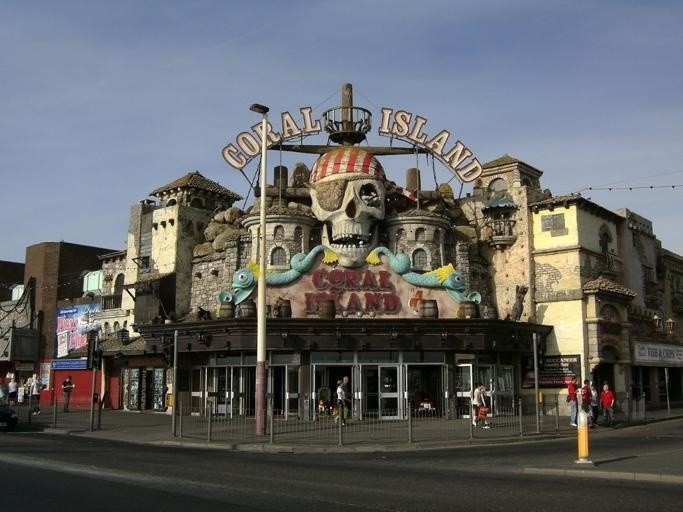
xmin=603 ymin=384 xmax=609 ymax=390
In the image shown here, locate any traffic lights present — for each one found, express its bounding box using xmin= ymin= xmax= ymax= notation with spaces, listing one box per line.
xmin=92 ymin=350 xmax=98 ymax=368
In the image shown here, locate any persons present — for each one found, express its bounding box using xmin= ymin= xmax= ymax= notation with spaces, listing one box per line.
xmin=103 ymin=320 xmax=130 ymax=346
xmin=0 ymin=373 xmax=41 ymax=422
xmin=335 ymin=376 xmax=353 ymax=426
xmin=62 ymin=375 xmax=74 ymax=413
xmin=568 ymin=376 xmax=615 ymax=427
xmin=472 ymin=383 xmax=491 ymax=429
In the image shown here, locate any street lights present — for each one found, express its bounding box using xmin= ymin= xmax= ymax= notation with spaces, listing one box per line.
xmin=248 ymin=103 xmax=269 ymax=435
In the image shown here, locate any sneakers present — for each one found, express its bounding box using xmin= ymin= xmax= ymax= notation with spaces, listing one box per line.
xmin=571 ymin=423 xmax=578 ymax=427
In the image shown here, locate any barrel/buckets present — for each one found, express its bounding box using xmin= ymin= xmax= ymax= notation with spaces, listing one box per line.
xmin=320 ymin=301 xmax=336 ymax=318
xmin=238 ymin=300 xmax=255 ymax=317
xmin=421 ymin=299 xmax=438 ymax=318
xmin=486 ymin=307 xmax=496 ymax=319
xmin=218 ymin=299 xmax=234 ymax=317
xmin=458 ymin=302 xmax=474 ymax=317
xmin=274 ymin=300 xmax=293 ymax=317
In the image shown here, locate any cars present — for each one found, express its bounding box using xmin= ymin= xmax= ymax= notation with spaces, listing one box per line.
xmin=0 ymin=399 xmax=20 ymax=430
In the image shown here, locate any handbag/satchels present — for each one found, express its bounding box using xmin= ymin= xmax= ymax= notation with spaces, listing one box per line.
xmin=566 ymin=396 xmax=571 ymax=403
xmin=480 ymin=407 xmax=489 ymax=420
